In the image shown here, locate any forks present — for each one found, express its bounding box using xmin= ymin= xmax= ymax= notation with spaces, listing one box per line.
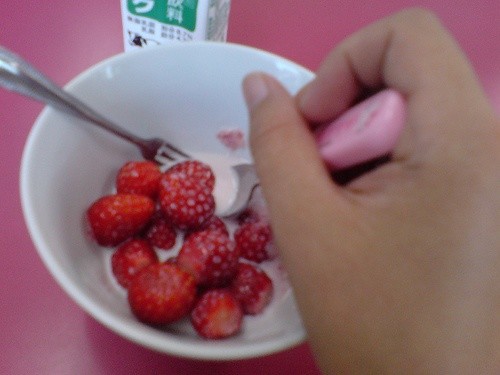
xmin=0 ymin=46 xmax=192 ymax=166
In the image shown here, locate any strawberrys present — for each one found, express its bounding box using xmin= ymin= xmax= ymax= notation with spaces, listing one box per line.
xmin=87 ymin=160 xmax=280 ymax=339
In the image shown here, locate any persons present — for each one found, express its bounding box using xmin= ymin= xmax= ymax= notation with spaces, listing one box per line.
xmin=243 ymin=8 xmax=500 ymax=375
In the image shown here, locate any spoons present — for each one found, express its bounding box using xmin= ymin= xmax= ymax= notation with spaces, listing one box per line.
xmin=220 ymin=88 xmax=408 ymax=217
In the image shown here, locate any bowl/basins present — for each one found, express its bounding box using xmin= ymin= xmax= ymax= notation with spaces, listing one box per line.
xmin=18 ymin=41 xmax=317 ymax=361
